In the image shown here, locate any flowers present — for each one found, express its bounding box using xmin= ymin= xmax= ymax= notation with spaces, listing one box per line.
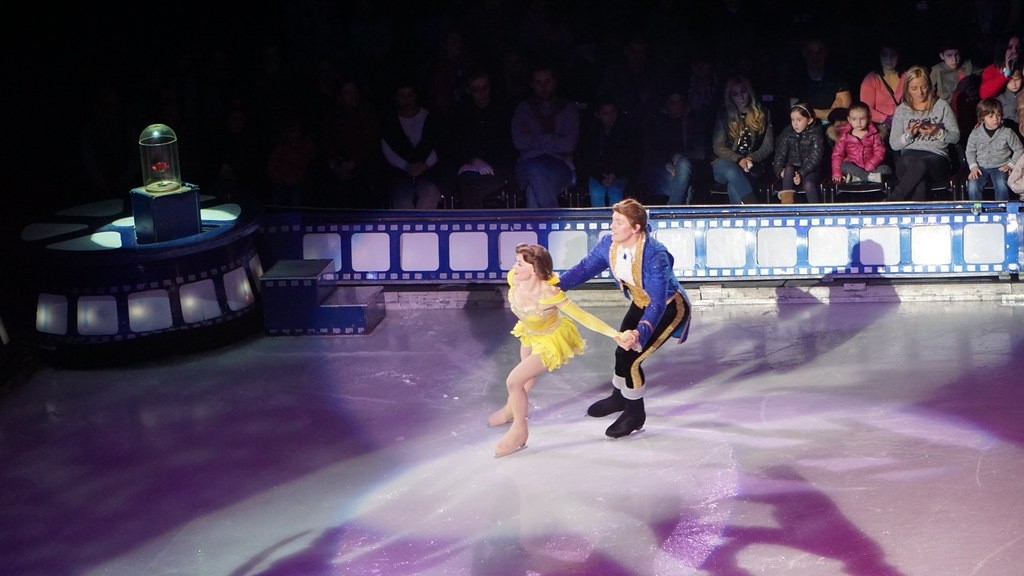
xmin=151 ymin=160 xmax=170 ymax=188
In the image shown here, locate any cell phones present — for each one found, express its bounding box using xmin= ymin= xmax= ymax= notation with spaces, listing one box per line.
xmin=744 ymin=162 xmax=753 ymax=172
xmin=920 ymin=121 xmax=930 ymax=129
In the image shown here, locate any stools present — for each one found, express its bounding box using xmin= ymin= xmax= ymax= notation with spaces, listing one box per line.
xmin=439 ymin=148 xmax=1024 ymax=208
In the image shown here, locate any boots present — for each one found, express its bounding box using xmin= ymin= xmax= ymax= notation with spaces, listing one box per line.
xmin=780 ymin=190 xmax=794 ymax=204
xmin=741 ymin=192 xmax=760 ymax=204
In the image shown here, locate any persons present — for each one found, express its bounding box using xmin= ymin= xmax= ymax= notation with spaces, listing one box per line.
xmin=1005 ymin=89 xmax=1024 ymax=203
xmin=830 ymin=101 xmax=893 ymax=185
xmin=774 ymin=103 xmax=826 ymax=205
xmin=376 ymin=84 xmax=447 ymax=212
xmin=438 ymin=70 xmax=513 ymax=206
xmin=964 ymin=96 xmax=1024 ymax=201
xmin=708 ymin=74 xmax=775 ymax=205
xmin=978 ymin=27 xmax=1024 ymax=101
xmin=860 ymin=41 xmax=912 ymax=140
xmin=271 ymin=114 xmax=320 ymax=207
xmin=320 ymin=79 xmax=387 ymax=210
xmin=883 ymin=65 xmax=962 ymax=204
xmin=575 ymin=96 xmax=632 ymax=207
xmin=992 ymin=62 xmax=1024 ymax=158
xmin=316 ymin=1 xmax=860 ymax=144
xmin=509 ymin=59 xmax=581 ymax=208
xmin=928 ymin=43 xmax=984 ymax=112
xmin=208 ymin=103 xmax=265 ymax=201
xmin=485 ymin=242 xmax=644 ymax=458
xmin=634 ymin=88 xmax=704 ymax=207
xmin=554 ymin=199 xmax=692 ymax=438
xmin=824 ymin=107 xmax=850 ymax=166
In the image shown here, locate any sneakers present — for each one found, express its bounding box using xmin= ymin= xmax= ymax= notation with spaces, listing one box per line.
xmin=496 ymin=421 xmax=529 ymax=458
xmin=488 ymin=395 xmax=514 ymax=426
xmin=585 ymin=387 xmax=625 ymax=418
xmin=603 ymin=398 xmax=646 ymax=441
